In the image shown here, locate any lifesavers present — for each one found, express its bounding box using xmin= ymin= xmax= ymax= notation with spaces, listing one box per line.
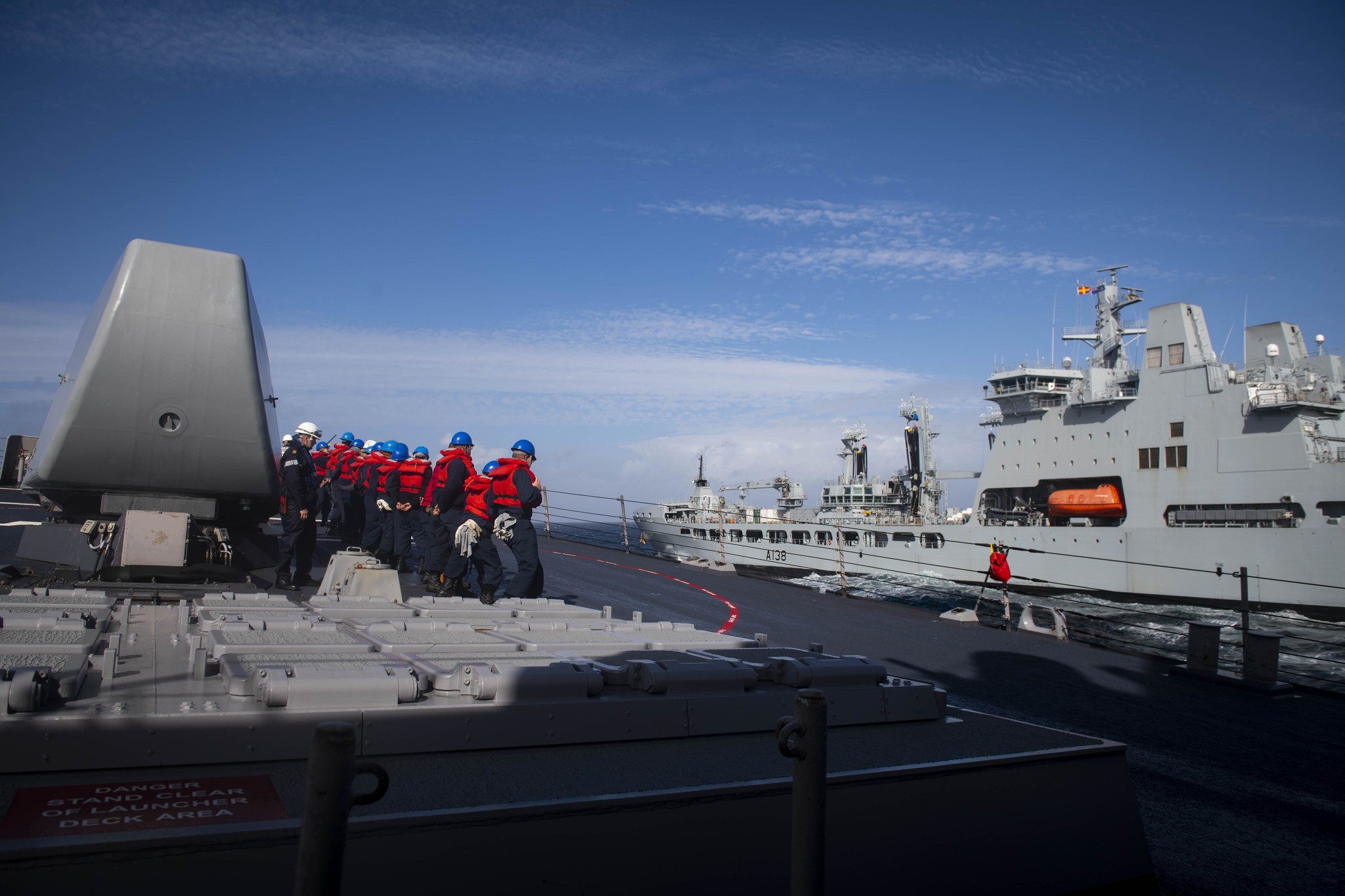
xmin=784 ymin=518 xmax=788 ymax=522
xmin=805 ymin=494 xmax=806 ymax=498
xmin=828 ymin=540 xmax=831 ymax=544
xmin=882 ymin=489 xmax=884 ymax=494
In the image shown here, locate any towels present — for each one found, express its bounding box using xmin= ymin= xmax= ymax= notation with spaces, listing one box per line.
xmin=496 ymin=512 xmax=517 ymax=542
xmin=455 ymin=526 xmax=478 ymax=558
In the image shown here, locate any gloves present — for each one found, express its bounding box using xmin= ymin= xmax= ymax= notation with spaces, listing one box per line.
xmin=376 ymin=499 xmax=391 ymax=511
xmin=492 ymin=512 xmax=508 ymax=540
xmin=463 ymin=519 xmax=482 ymax=539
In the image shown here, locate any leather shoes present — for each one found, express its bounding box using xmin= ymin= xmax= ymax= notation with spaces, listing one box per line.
xmin=292 ymin=571 xmax=320 ymax=586
xmin=275 ymin=576 xmax=299 ymax=590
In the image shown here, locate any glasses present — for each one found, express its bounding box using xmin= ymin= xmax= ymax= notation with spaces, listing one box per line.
xmin=297 ymin=427 xmax=322 ymax=437
xmin=467 ymin=446 xmax=474 ymax=450
xmin=524 ymin=456 xmax=535 ymax=463
xmin=322 ymin=448 xmax=330 ymax=451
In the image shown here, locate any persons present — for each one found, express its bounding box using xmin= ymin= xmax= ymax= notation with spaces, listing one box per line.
xmin=275 ymin=422 xmax=320 ymax=590
xmin=460 ymin=461 xmax=504 ymax=605
xmin=388 ymin=446 xmax=432 ymax=574
xmin=690 ymin=516 xmax=696 ymax=523
xmin=681 ymin=515 xmax=684 ymax=523
xmin=281 ymin=434 xmax=293 ymax=457
xmin=488 ymin=439 xmax=544 ymax=598
xmin=421 ymin=432 xmax=477 ymax=597
xmin=309 ymin=433 xmax=412 ymax=558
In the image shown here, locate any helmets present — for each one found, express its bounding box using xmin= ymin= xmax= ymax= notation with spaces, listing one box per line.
xmin=450 ymin=431 xmax=475 ymax=446
xmin=482 ymin=460 xmax=500 ymax=473
xmin=412 ymin=446 xmax=429 ymax=460
xmin=511 ymin=439 xmax=537 ymax=460
xmin=282 ymin=434 xmax=293 ymax=443
xmin=350 ymin=439 xmax=365 ymax=448
xmin=339 ymin=432 xmax=355 ymax=442
xmin=380 ymin=440 xmax=411 ymax=460
xmin=362 ymin=440 xmax=385 ymax=455
xmin=295 ymin=422 xmax=321 ymax=440
xmin=316 ymin=442 xmax=330 ymax=451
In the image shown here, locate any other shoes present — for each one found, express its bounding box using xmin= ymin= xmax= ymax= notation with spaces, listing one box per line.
xmin=371 ymin=549 xmax=389 ymax=565
xmin=324 ymin=527 xmax=337 ymax=537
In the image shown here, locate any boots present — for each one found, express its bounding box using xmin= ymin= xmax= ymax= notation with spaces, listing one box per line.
xmin=320 ymin=516 xmax=332 ymax=526
xmin=420 ymin=571 xmax=430 ymax=584
xmin=416 ymin=558 xmax=424 ymax=574
xmin=479 ymin=584 xmax=495 ymax=606
xmin=395 ymin=557 xmax=414 ymax=574
xmin=427 ymin=574 xmax=443 ymax=595
xmin=436 ymin=576 xmax=478 ymax=600
